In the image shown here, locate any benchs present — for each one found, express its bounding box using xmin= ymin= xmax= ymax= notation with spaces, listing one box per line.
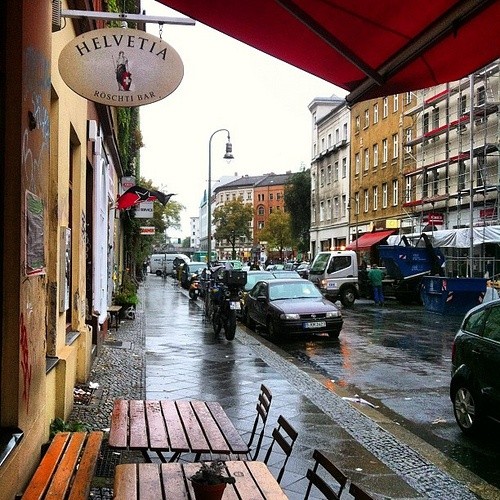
xmin=20 ymin=431 xmax=103 ymax=500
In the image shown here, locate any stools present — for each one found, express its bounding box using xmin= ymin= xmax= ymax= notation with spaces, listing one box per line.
xmin=108 ymin=305 xmax=122 ymax=331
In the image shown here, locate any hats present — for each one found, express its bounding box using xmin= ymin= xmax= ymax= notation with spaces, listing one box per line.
xmin=373 ymin=264 xmax=378 ymax=268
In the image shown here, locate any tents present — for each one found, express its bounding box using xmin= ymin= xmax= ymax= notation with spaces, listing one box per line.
xmin=387 ymin=225 xmax=500 ymax=250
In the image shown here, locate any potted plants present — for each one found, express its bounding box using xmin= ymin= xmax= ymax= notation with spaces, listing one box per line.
xmin=188 ymin=458 xmax=236 ymax=500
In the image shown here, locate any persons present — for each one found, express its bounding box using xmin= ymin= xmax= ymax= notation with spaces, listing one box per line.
xmin=248 ymin=256 xmax=310 ymax=270
xmin=368 ymin=264 xmax=386 ymax=307
xmin=142 ymin=261 xmax=149 ymax=276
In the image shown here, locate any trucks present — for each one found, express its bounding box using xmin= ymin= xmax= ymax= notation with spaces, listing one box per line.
xmin=307 ymin=249 xmax=422 ymax=308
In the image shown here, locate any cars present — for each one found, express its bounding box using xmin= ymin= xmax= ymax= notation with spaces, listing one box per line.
xmin=450 ymin=298 xmax=500 ymax=435
xmin=243 ymin=277 xmax=344 ymax=343
xmin=178 ymin=249 xmax=311 ymax=293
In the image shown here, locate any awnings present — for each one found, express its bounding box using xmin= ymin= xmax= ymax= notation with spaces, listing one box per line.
xmin=346 ymin=230 xmax=397 ymax=251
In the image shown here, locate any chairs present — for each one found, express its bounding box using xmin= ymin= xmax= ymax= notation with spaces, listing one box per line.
xmin=246 ymin=383 xmax=375 ymax=500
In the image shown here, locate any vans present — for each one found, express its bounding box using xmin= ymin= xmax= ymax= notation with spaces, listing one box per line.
xmin=149 ymin=253 xmax=193 ymax=276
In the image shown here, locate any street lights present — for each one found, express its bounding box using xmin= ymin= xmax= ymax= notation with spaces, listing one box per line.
xmin=346 ymin=196 xmax=360 ymax=267
xmin=205 ymin=128 xmax=235 ymax=318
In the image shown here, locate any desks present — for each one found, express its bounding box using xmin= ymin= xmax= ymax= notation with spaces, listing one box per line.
xmin=109 ymin=399 xmax=288 ymax=500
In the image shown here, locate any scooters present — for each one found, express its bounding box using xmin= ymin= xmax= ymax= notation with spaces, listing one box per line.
xmin=188 ymin=266 xmax=248 ymax=341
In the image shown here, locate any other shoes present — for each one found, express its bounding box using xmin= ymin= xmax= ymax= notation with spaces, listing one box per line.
xmin=373 ymin=303 xmax=379 ymax=305
xmin=381 ymin=304 xmax=384 ymax=306
